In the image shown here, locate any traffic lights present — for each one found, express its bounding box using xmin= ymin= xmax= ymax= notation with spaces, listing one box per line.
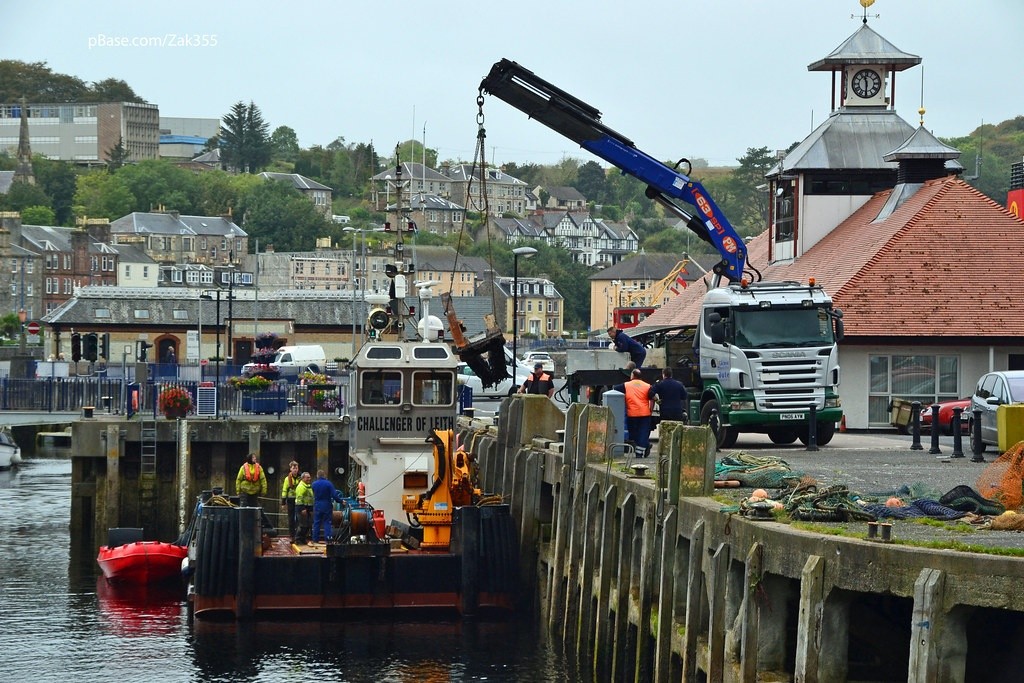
xmin=99 ymin=334 xmax=106 ymax=359
xmin=141 ymin=340 xmax=153 ymax=361
xmin=87 ymin=333 xmax=98 ymax=360
xmin=369 ymin=329 xmax=376 ymax=340
xmin=71 ymin=333 xmax=81 ymax=362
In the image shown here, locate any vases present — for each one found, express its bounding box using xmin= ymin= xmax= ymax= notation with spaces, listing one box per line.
xmin=164 ymin=407 xmax=186 ymax=419
xmin=242 ymin=391 xmax=287 ymax=415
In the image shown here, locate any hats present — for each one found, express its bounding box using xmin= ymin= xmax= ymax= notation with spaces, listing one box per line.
xmin=533 ymin=364 xmax=542 ymax=369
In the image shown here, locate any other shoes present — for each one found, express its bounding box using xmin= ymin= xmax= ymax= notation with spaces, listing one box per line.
xmin=645 ymin=444 xmax=652 ymax=458
xmin=636 ymin=453 xmax=642 ymax=458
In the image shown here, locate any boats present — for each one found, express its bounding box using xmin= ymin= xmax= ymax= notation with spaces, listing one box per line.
xmin=0 ymin=424 xmax=19 ymax=470
xmin=97 ymin=540 xmax=188 ymax=588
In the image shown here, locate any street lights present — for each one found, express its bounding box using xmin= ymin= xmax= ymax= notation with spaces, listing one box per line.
xmin=511 ymin=247 xmax=538 ymax=393
xmin=199 ymin=288 xmax=236 ymax=419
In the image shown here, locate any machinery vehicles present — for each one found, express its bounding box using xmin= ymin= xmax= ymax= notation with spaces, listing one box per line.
xmin=484 ymin=59 xmax=846 ymax=449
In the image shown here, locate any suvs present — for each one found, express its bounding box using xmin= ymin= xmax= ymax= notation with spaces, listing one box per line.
xmin=520 ymin=351 xmax=555 ymax=381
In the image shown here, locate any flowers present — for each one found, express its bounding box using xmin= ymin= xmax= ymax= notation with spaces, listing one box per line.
xmin=229 ymin=377 xmax=269 ymax=392
xmin=301 ymin=371 xmax=343 ymax=407
xmin=160 ymin=386 xmax=193 ymax=412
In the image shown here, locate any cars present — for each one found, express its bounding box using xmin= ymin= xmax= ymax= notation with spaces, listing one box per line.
xmin=456 ymin=361 xmax=530 ymax=399
xmin=969 ymin=370 xmax=1024 ymax=455
xmin=920 ymin=395 xmax=973 ymax=436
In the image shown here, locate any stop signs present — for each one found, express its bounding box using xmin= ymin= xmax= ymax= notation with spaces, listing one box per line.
xmin=27 ymin=322 xmax=40 ymax=335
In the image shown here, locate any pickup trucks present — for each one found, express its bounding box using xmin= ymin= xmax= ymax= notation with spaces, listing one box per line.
xmin=241 ymin=345 xmax=326 ymax=379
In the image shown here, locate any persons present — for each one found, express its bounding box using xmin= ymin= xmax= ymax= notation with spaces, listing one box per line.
xmin=649 ymin=366 xmax=688 ymax=421
xmin=295 ymin=471 xmax=314 ymax=545
xmin=607 ymin=326 xmax=657 ymax=369
xmin=312 ymin=470 xmax=347 ymax=545
xmin=281 ymin=461 xmax=301 ymax=544
xmin=387 ymin=391 xmax=400 ymax=404
xmin=613 ymin=361 xmax=655 ymax=458
xmin=517 ymin=362 xmax=555 ymax=398
xmin=166 ymin=346 xmax=175 ymax=368
xmin=621 ymin=316 xmax=633 ymax=323
xmin=236 ymin=452 xmax=267 ymax=507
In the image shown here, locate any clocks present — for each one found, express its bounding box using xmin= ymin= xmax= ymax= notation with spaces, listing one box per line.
xmin=851 ymin=69 xmax=881 ymax=99
